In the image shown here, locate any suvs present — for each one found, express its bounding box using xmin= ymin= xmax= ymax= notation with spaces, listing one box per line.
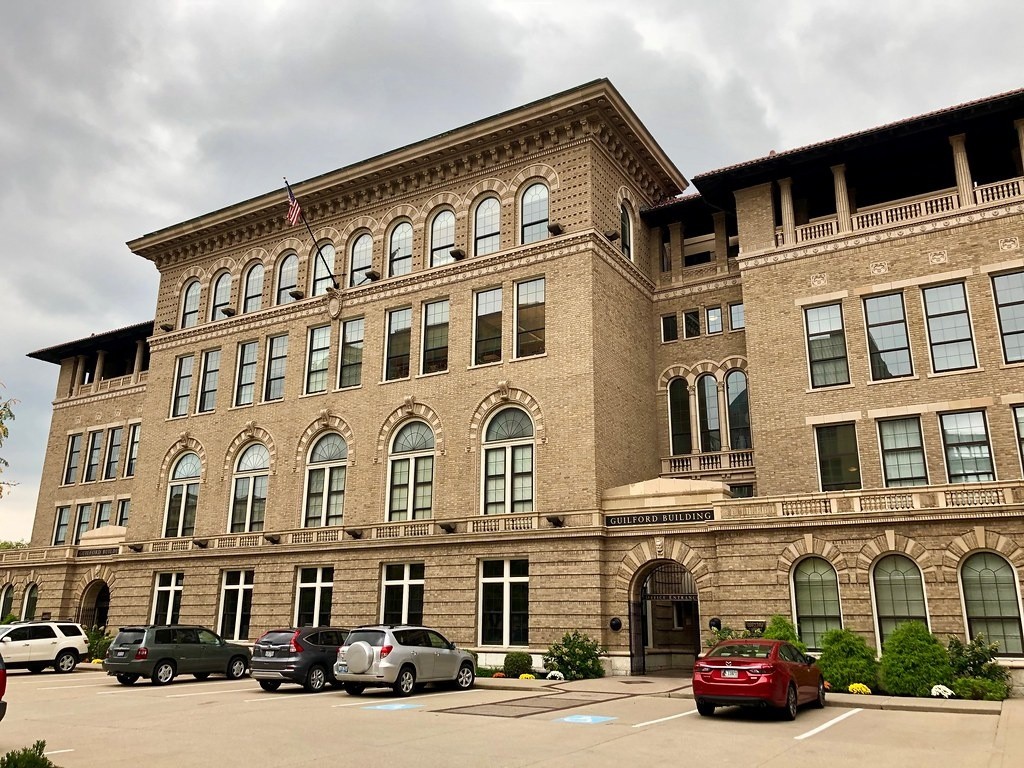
xmin=0 ymin=620 xmax=91 ymax=674
xmin=332 ymin=623 xmax=478 ymax=696
xmin=101 ymin=623 xmax=252 ymax=686
xmin=249 ymin=626 xmax=353 ymax=693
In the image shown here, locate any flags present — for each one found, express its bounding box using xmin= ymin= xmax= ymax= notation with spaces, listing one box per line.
xmin=284 ymin=181 xmax=301 ymax=226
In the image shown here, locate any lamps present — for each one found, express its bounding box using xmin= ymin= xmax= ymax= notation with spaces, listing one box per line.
xmin=193 ymin=540 xmax=209 ymax=548
xmin=449 ymin=249 xmax=465 ymax=261
xmin=438 ymin=521 xmax=457 ymax=534
xmin=547 ymin=223 xmax=563 ymax=236
xmin=365 ymin=270 xmax=381 ymax=282
xmin=345 ymin=529 xmax=363 ymax=540
xmin=160 ymin=324 xmax=173 ymax=332
xmin=221 ymin=308 xmax=236 ymax=318
xmin=604 ymin=230 xmax=621 ymax=242
xmin=546 ymin=514 xmax=565 ymax=528
xmin=263 ymin=534 xmax=280 ymax=544
xmin=290 ymin=291 xmax=304 ymax=300
xmin=128 ymin=544 xmax=144 ymax=552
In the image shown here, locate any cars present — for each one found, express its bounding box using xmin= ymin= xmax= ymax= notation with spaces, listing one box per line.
xmin=692 ymin=638 xmax=825 ymax=721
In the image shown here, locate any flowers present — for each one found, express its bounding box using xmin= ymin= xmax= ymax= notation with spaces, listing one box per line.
xmin=930 ymin=684 xmax=956 ymax=699
xmin=519 ymin=673 xmax=535 ymax=679
xmin=824 ymin=681 xmax=832 ymax=692
xmin=493 ymin=672 xmax=506 ymax=678
xmin=848 ymin=682 xmax=872 ymax=695
xmin=546 ymin=671 xmax=564 ymax=681
xmin=91 ymin=658 xmax=103 ymax=664
